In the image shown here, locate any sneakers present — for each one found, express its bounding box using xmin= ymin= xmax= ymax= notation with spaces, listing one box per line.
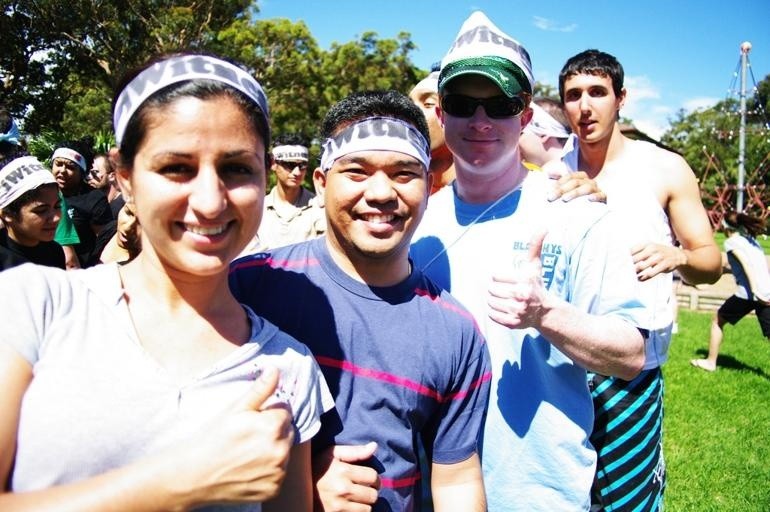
xmin=672 ymin=322 xmax=679 ymax=332
xmin=690 ymin=358 xmax=717 ymax=372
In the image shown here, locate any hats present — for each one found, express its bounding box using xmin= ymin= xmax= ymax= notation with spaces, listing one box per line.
xmin=435 ymin=9 xmax=537 ymax=99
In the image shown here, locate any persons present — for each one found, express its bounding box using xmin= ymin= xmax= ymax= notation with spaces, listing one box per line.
xmin=518 ymin=98 xmax=574 ymax=169
xmin=541 ymin=49 xmax=723 ymax=511
xmin=0 ymin=110 xmax=81 ymax=270
xmin=86 ymin=202 xmax=144 ymax=266
xmin=409 ymin=11 xmax=648 ymax=511
xmin=108 ymin=143 xmax=120 ymax=167
xmin=86 ymin=151 xmax=125 ymax=237
xmin=51 ymin=135 xmax=111 ymax=267
xmin=0 ymin=49 xmax=336 ymax=512
xmin=229 ymin=90 xmax=493 ymax=512
xmin=407 ymin=65 xmax=607 ymax=205
xmin=236 ymin=132 xmax=326 ymax=257
xmin=1 ymin=151 xmax=66 ymax=269
xmin=689 ymin=211 xmax=770 ymax=372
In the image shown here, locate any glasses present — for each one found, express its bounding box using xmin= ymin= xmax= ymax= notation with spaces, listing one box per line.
xmin=441 ymin=92 xmax=524 ymax=120
xmin=276 ymin=159 xmax=308 ymax=171
